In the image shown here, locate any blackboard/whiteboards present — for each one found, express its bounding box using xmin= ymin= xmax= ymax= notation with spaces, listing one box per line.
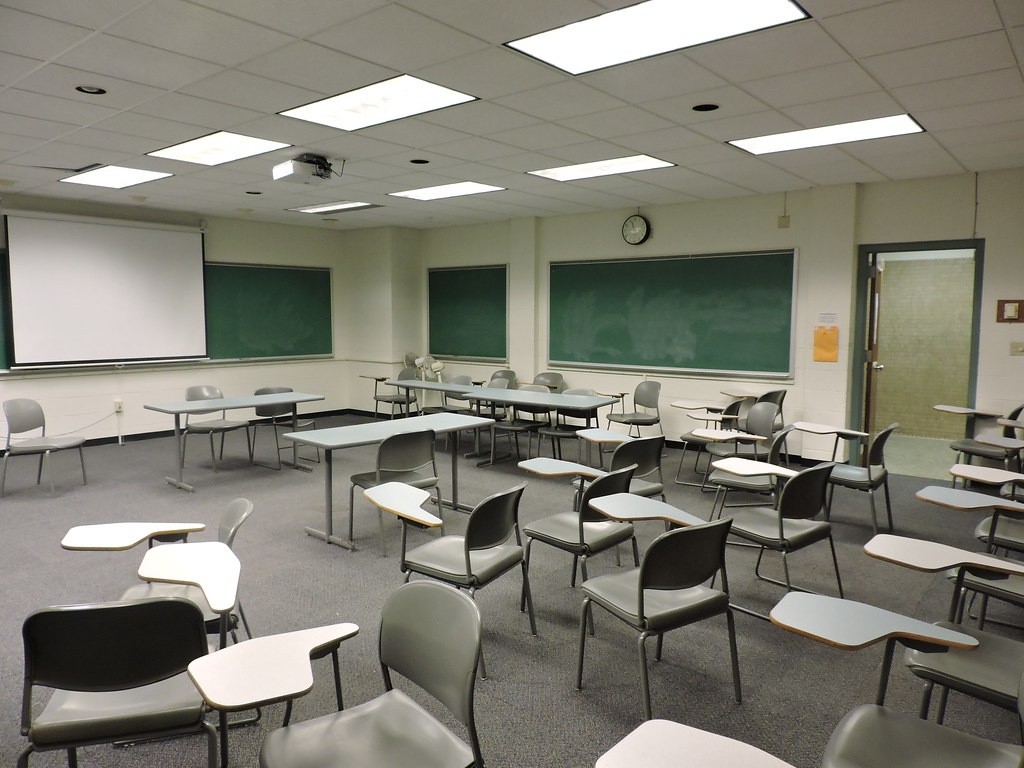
xmin=426 ymin=264 xmax=510 ymax=362
xmin=548 ymin=247 xmax=800 ymax=380
xmin=0 ymin=247 xmax=334 ymax=374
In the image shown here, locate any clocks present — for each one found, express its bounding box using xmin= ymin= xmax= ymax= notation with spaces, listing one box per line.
xmin=622 ymin=215 xmax=650 ymax=245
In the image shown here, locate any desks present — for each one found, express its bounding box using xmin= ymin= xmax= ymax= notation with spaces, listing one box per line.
xmin=143 ymin=392 xmax=325 ymax=492
xmin=282 ymin=412 xmax=496 ymax=552
xmin=461 ymin=389 xmax=620 ymax=469
xmin=384 ymin=380 xmax=491 ymax=458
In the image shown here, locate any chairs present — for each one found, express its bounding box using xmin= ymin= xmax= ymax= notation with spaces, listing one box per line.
xmin=0 ymin=353 xmax=1024 ymax=768
